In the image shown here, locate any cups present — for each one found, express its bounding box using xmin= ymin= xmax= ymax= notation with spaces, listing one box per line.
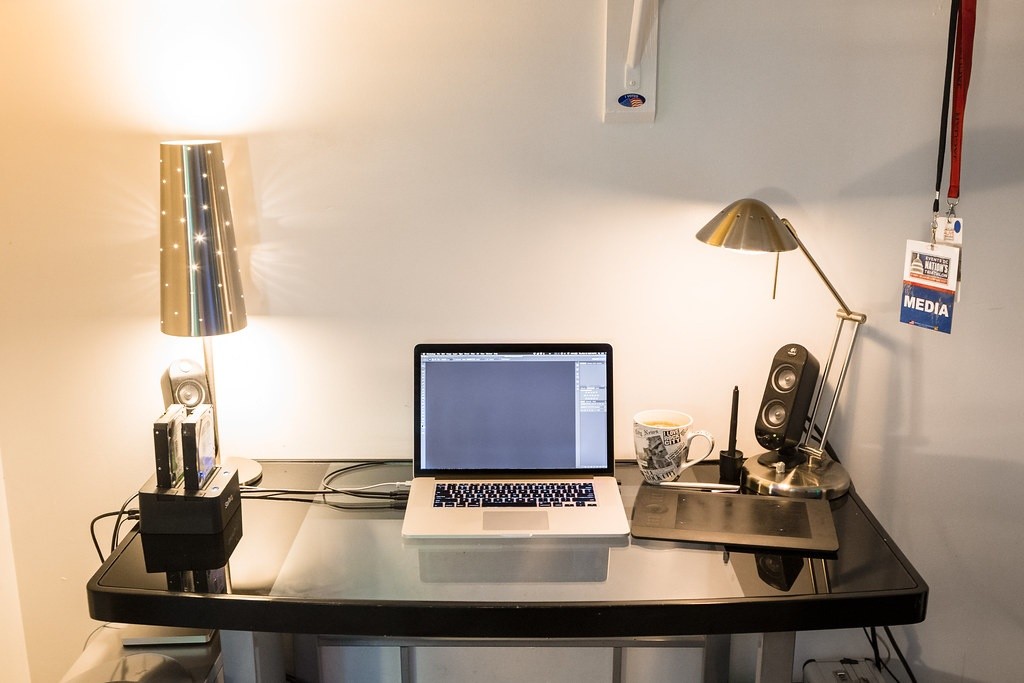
xmin=633 ymin=409 xmax=714 ymax=486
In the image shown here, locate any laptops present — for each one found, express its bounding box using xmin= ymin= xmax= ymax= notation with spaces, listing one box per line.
xmin=400 ymin=342 xmax=629 ymax=540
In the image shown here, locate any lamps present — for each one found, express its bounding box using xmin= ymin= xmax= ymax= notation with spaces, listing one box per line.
xmin=694 ymin=197 xmax=868 ymax=504
xmin=157 ymin=139 xmax=266 ymax=485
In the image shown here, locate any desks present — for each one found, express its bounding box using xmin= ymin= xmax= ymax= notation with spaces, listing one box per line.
xmin=86 ymin=457 xmax=928 ymax=683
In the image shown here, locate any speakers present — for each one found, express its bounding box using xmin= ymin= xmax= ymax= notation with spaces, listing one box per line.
xmin=755 ymin=342 xmax=819 ymax=467
xmin=755 ymin=555 xmax=805 ymax=593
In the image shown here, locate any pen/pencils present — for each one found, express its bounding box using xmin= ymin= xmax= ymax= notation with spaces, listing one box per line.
xmin=659 ymin=481 xmax=741 ymax=493
xmin=728 ymin=385 xmax=739 ymax=457
